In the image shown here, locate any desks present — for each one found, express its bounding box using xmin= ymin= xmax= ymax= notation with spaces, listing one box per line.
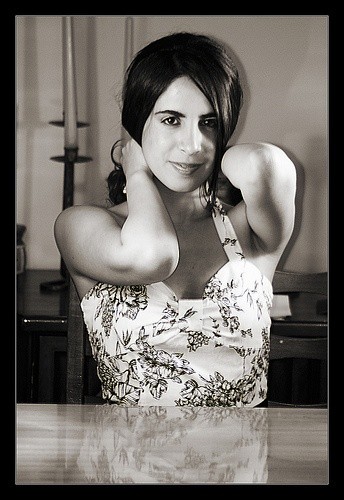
xmin=16 ymin=404 xmax=329 ymax=484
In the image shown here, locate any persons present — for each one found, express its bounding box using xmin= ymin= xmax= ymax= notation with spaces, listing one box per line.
xmin=70 ymin=406 xmax=272 ymax=486
xmin=52 ymin=31 xmax=296 ymax=407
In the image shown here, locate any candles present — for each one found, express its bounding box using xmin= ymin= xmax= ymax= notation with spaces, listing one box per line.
xmin=61 ymin=16 xmax=77 ymax=148
xmin=118 ymin=16 xmax=134 ymax=165
